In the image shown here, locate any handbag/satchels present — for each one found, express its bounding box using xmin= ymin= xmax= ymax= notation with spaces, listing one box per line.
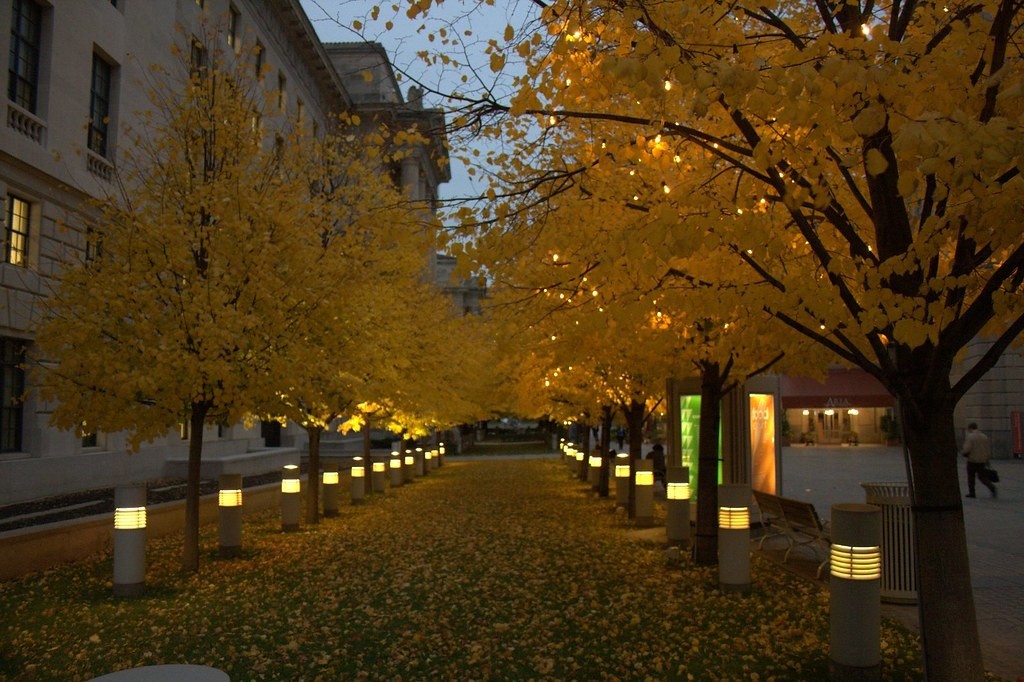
xmin=986 ymin=464 xmax=999 ymax=483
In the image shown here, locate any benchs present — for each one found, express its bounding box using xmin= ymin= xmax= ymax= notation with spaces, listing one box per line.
xmin=751 ymin=489 xmax=832 ymax=580
xmin=644 ymin=460 xmax=667 ymax=492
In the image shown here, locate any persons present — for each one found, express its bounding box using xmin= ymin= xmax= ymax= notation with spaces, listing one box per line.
xmin=961 ymin=423 xmax=1000 ymax=497
xmin=647 ymin=444 xmax=668 ymax=489
xmin=617 ymin=427 xmax=625 ymax=449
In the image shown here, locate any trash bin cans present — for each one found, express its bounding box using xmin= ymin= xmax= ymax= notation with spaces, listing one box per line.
xmin=860 ymin=480 xmax=919 ymax=607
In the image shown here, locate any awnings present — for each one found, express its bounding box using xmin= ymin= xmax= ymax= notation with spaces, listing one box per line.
xmin=780 ymin=369 xmax=899 ymax=408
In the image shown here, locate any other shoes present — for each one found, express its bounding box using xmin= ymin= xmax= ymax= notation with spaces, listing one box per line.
xmin=966 ymin=494 xmax=976 ymax=498
xmin=991 ymin=487 xmax=996 ymax=496
